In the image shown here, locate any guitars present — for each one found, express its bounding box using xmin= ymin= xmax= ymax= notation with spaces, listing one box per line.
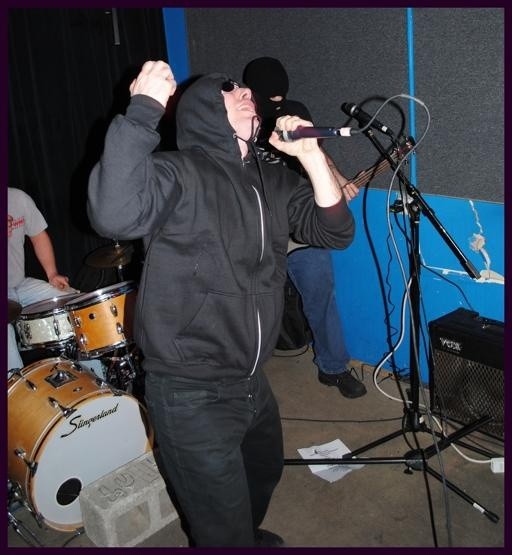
xmin=287 ymin=139 xmax=413 ymax=254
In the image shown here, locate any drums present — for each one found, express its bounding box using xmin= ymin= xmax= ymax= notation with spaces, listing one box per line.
xmin=64 ymin=280 xmax=142 ymax=361
xmin=16 ymin=293 xmax=86 ymax=349
xmin=7 ymin=356 xmax=155 ymax=533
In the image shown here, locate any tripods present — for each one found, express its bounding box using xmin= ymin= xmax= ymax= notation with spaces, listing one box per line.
xmin=284 ymin=126 xmax=503 ymax=525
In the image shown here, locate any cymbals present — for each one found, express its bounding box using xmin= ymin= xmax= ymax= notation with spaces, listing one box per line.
xmin=7 ymin=298 xmax=24 ymax=325
xmin=84 ymin=241 xmax=144 ymax=268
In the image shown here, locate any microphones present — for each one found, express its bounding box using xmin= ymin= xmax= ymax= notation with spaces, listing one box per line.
xmin=340 ymin=100 xmax=394 ymax=136
xmin=274 ymin=125 xmax=360 ymax=143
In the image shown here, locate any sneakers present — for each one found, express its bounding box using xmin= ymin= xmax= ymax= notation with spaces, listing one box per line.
xmin=318 ymin=367 xmax=367 ymax=399
xmin=255 ymin=528 xmax=286 ymax=548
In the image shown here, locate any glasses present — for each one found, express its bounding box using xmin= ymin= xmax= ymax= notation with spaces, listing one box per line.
xmin=221 ymin=79 xmax=239 ymax=92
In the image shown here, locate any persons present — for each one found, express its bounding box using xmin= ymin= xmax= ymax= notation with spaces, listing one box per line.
xmin=245 ymin=59 xmax=370 ymax=399
xmin=7 ymin=188 xmax=108 ymax=380
xmin=84 ymin=60 xmax=355 ymax=550
